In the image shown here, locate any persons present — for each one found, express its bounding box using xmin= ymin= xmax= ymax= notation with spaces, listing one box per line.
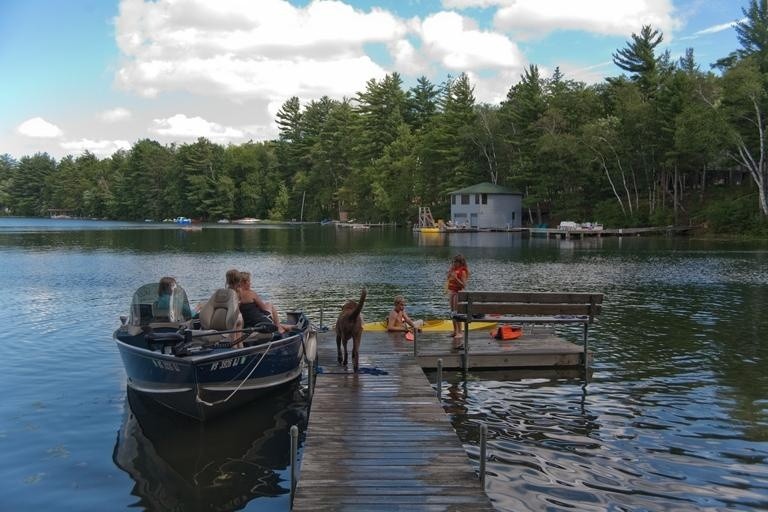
xmin=225 ymin=269 xmax=244 ymax=349
xmin=237 ymin=272 xmax=288 ymax=334
xmin=388 ymin=295 xmax=421 ymax=333
xmin=447 ymin=255 xmax=468 ymax=339
xmin=153 ymin=277 xmax=202 ymax=321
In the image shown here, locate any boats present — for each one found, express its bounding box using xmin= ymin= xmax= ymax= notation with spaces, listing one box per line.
xmin=419 ymin=227 xmax=443 ymax=233
xmin=217 ymin=216 xmax=262 ymax=225
xmin=113 ymin=282 xmax=317 ymax=422
xmin=163 ymin=216 xmax=201 ymax=225
xmin=361 ymin=319 xmax=498 ymax=333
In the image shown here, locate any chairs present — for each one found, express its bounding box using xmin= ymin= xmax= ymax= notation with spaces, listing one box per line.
xmin=153 ymin=277 xmax=191 ymax=321
xmin=199 ymin=287 xmax=245 ymax=350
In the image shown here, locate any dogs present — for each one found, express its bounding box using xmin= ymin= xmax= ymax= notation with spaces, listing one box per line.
xmin=335 ymin=287 xmax=367 ymax=373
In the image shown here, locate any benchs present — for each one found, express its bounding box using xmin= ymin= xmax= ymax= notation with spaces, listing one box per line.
xmin=451 ymin=290 xmax=603 ymax=371
